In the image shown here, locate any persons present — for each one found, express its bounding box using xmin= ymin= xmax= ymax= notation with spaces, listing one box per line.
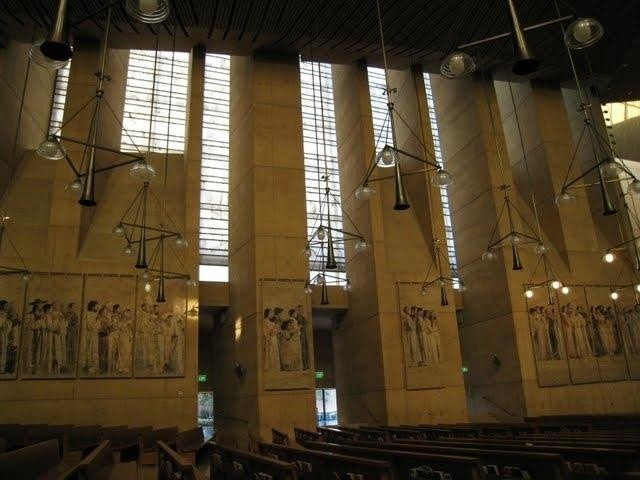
xmin=264 ymin=305 xmax=308 ymax=374
xmin=529 ymin=303 xmax=640 ymax=361
xmin=402 ymin=307 xmax=440 ymax=369
xmin=0 ymin=299 xmax=184 ymax=375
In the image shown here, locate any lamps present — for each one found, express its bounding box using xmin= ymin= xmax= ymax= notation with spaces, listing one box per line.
xmin=304 ymin=42 xmax=350 ymax=306
xmin=300 ymin=0 xmax=472 ymax=310
xmin=303 ymin=8 xmax=366 ymax=269
xmin=364 ymin=2 xmax=441 ymax=211
xmin=481 ymin=68 xmax=542 ymax=271
xmin=436 ymin=1 xmax=640 ymax=303
xmin=36 ymin=3 xmax=155 ymax=209
xmin=408 ymin=58 xmax=463 ymax=306
xmin=110 ymin=1 xmax=196 ymax=302
xmin=554 ymin=4 xmax=640 ymax=215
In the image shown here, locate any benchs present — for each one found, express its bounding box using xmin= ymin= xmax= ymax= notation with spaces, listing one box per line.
xmin=0 ymin=420 xmax=640 ymax=480
